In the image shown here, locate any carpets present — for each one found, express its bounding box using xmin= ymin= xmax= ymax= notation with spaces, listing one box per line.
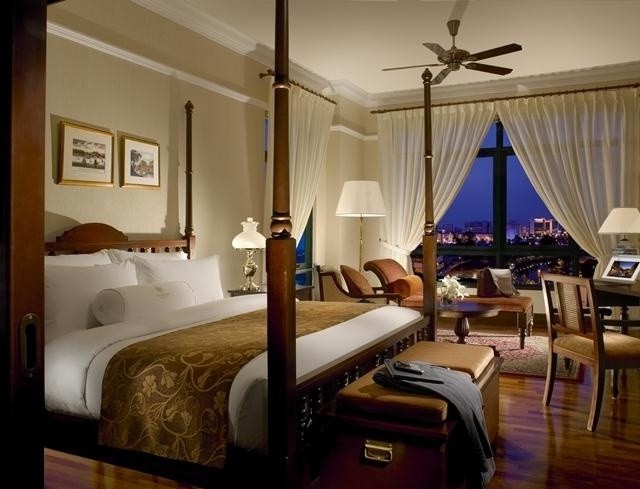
xmin=414 ymin=330 xmax=581 ymax=380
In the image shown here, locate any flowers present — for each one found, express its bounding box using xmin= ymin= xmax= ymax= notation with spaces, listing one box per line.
xmin=438 ymin=273 xmax=470 ymax=300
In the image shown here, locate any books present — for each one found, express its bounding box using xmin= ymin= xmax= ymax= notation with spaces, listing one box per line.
xmin=382 ymin=357 xmax=444 ymax=386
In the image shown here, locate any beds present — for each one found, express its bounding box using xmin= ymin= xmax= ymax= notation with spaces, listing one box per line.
xmin=44 ymin=1 xmax=434 ymax=489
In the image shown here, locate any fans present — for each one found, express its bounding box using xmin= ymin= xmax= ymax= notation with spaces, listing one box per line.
xmin=382 ymin=20 xmax=522 ymax=85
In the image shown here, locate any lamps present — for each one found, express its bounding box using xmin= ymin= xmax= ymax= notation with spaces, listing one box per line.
xmin=598 ymin=208 xmax=640 ymax=255
xmin=231 ymin=218 xmax=268 ymax=292
xmin=335 ymin=180 xmax=387 ymax=273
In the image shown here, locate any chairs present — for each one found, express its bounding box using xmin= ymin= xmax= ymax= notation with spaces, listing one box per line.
xmin=537 ymin=269 xmax=640 ymax=432
xmin=315 ymin=259 xmax=423 ymax=307
xmin=476 ymin=270 xmax=523 ymax=337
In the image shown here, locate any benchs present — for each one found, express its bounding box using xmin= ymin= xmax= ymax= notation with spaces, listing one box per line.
xmin=328 ymin=342 xmax=504 ymax=489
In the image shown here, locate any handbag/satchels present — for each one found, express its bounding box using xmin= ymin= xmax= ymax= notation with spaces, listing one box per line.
xmin=477 ymin=269 xmax=511 ymax=298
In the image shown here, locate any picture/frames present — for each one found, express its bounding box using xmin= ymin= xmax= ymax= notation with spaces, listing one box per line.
xmin=601 ymin=256 xmax=640 ymax=281
xmin=118 ymin=135 xmax=160 ymax=190
xmin=56 ymin=120 xmax=114 ymax=187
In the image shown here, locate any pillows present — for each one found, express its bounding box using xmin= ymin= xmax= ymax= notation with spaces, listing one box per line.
xmin=44 ymin=248 xmax=224 ymax=344
